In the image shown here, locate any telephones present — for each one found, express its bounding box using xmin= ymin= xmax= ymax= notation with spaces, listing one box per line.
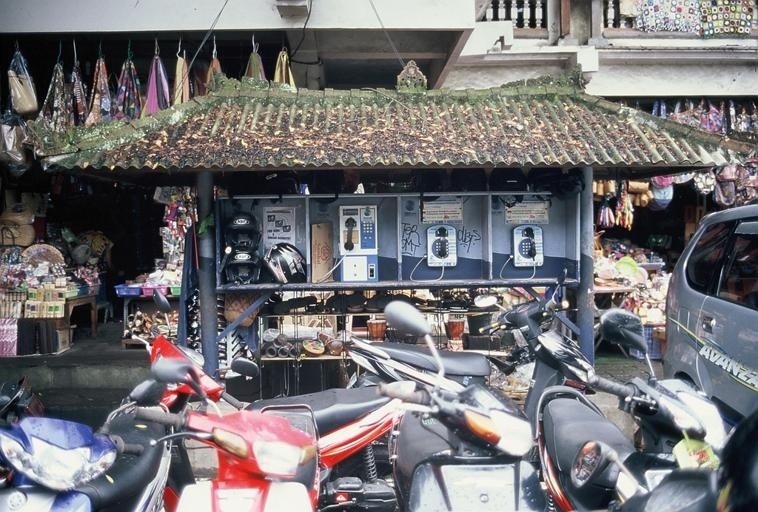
xmin=338 ymin=205 xmax=378 ymax=256
xmin=513 ymin=225 xmax=544 ymax=267
xmin=426 ymin=224 xmax=457 ymax=267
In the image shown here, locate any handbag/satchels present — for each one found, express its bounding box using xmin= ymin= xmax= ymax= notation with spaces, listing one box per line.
xmin=8 ymin=68 xmax=38 ymax=114
xmin=1 ymin=118 xmax=35 ymax=165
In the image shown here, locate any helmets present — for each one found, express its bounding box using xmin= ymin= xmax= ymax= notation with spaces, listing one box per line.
xmin=223 ymin=211 xmax=262 ymax=252
xmin=262 ymin=243 xmax=307 ymax=284
xmin=225 ymin=252 xmax=260 ymax=284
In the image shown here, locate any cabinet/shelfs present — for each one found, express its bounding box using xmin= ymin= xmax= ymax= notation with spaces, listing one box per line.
xmin=255 ymin=309 xmax=528 ymax=404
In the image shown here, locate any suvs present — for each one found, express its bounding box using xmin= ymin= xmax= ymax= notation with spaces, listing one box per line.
xmin=661 ymin=203 xmax=756 ymax=437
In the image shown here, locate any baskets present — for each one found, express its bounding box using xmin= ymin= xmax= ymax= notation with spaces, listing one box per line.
xmin=115 ymin=284 xmax=141 ymax=297
xmin=142 ymin=285 xmax=169 ymax=297
xmin=171 ymin=285 xmax=181 ymax=295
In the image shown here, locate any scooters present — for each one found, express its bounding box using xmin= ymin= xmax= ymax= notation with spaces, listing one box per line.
xmin=1 ymin=269 xmax=756 ymax=511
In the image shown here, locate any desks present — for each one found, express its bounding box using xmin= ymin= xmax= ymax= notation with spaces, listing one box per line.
xmin=120 ymin=291 xmax=180 ymax=350
xmin=594 ymin=285 xmax=640 ymax=358
xmin=62 ymin=292 xmax=101 ymax=340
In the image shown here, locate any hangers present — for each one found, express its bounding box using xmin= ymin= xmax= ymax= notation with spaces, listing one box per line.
xmin=3 ymin=35 xmax=264 ymax=62
